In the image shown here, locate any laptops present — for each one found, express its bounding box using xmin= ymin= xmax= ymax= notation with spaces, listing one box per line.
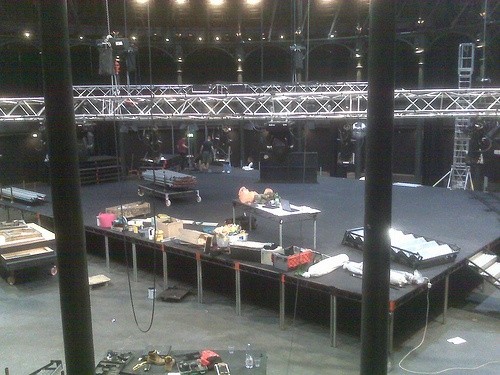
xmin=254 ymin=194 xmax=265 ymax=204
xmin=280 ymin=199 xmax=299 ymax=212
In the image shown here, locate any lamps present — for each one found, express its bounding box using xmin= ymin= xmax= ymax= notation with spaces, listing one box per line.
xmin=266 ymin=122 xmax=296 ymax=156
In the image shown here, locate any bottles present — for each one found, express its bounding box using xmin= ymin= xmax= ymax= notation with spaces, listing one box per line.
xmin=246 ymin=344 xmax=254 ymax=369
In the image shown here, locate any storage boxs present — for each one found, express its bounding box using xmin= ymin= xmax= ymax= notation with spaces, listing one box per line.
xmin=260 ymin=247 xmax=310 ymax=271
xmin=152 ymin=216 xmax=183 ymax=237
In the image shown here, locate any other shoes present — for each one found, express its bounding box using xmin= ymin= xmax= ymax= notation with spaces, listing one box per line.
xmin=221 ymin=170 xmax=225 ymax=172
xmin=227 ymin=171 xmax=231 ymax=173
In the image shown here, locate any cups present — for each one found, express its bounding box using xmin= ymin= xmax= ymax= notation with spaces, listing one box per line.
xmin=228 ymin=346 xmax=234 ymax=354
xmin=254 ymin=358 xmax=261 ymax=368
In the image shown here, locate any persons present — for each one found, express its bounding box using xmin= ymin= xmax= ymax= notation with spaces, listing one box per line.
xmin=221 ymin=142 xmax=232 ymax=173
xmin=463 ymin=124 xmax=488 ymax=192
xmin=199 ymin=136 xmax=216 ymax=170
xmin=176 ymin=136 xmax=189 ymax=173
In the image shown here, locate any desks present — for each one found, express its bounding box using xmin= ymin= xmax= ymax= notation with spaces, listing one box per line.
xmin=232 ymin=198 xmax=321 ymax=252
